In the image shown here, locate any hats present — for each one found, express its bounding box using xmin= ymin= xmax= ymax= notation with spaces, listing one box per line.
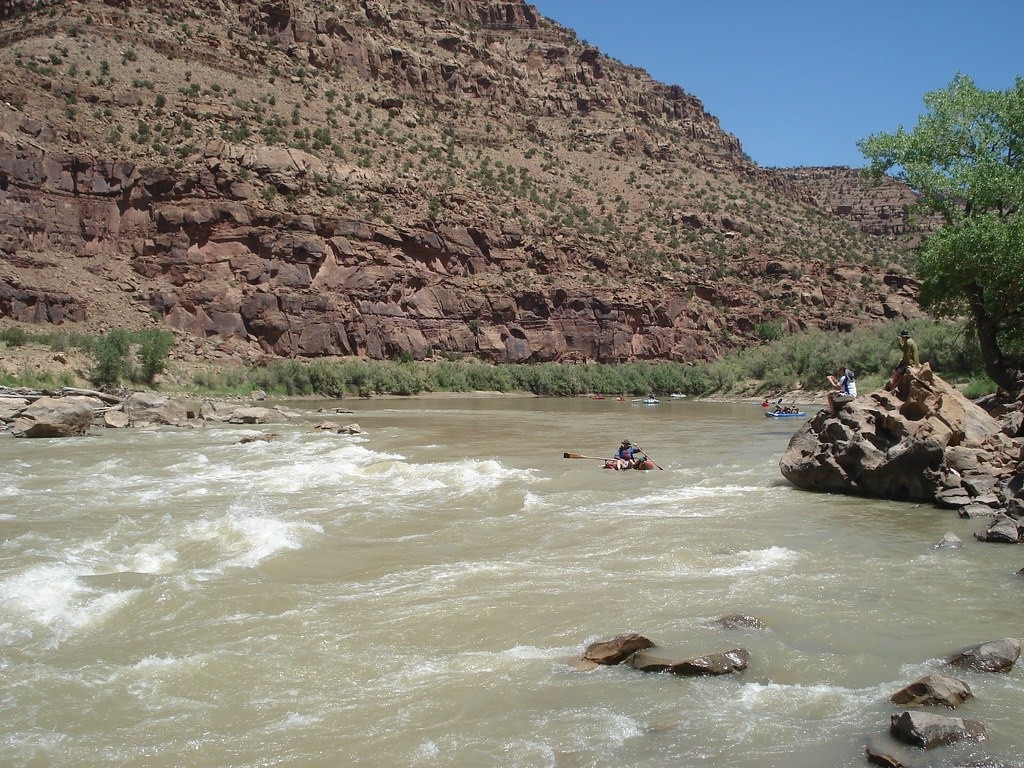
xmin=621 ymin=439 xmax=631 ymax=445
xmin=845 ymin=369 xmax=854 ymax=382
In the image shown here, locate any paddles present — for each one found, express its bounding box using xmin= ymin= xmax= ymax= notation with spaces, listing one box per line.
xmin=770 ymin=398 xmax=783 ymax=411
xmin=563 ymin=453 xmax=620 ymax=462
xmin=789 ymin=400 xmax=796 ymax=408
xmin=634 ymin=443 xmax=663 ymax=471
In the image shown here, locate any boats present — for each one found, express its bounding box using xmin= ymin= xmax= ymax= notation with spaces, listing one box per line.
xmin=631 ymin=399 xmax=641 ymax=403
xmin=592 ymin=393 xmax=604 ymax=400
xmin=617 ymin=396 xmax=624 ymax=402
xmin=643 ymin=394 xmax=659 ymax=404
xmin=765 ymin=411 xmax=805 ymax=417
xmin=603 ymin=460 xmax=653 ymax=470
xmin=670 ymin=392 xmax=686 ymax=397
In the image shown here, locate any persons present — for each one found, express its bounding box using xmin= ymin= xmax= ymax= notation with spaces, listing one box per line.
xmin=791 ymin=406 xmax=799 ymax=414
xmin=648 ymin=392 xmax=655 ymax=399
xmin=825 ymin=366 xmax=857 ymax=419
xmin=765 ymin=399 xmax=769 ymax=403
xmin=886 ymin=329 xmax=919 ymax=389
xmin=773 ymin=404 xmax=782 ymax=414
xmin=783 ymin=406 xmax=791 ymax=413
xmin=614 ymin=439 xmax=643 ymax=471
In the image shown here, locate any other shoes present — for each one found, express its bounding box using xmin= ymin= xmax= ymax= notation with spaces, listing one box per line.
xmin=826 ymin=414 xmax=836 ymax=418
